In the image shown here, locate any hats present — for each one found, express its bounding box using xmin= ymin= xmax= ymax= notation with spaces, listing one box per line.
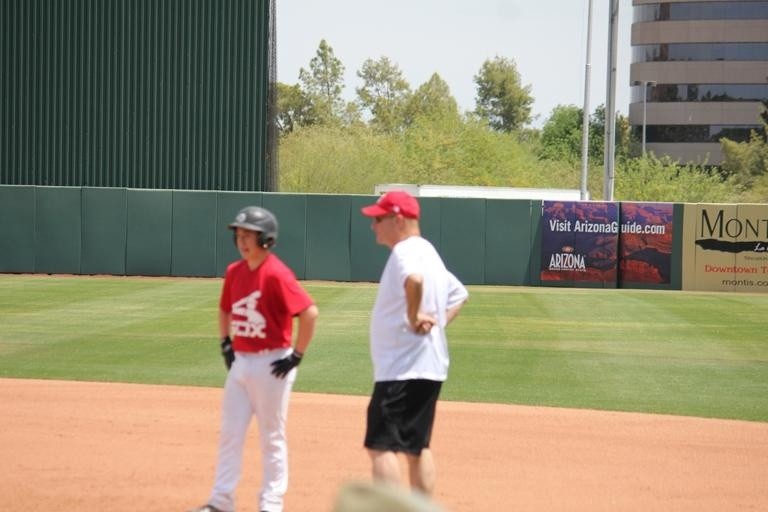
xmin=360 ymin=189 xmax=420 ymax=219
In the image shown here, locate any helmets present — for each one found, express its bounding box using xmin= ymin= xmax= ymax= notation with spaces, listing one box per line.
xmin=227 ymin=205 xmax=279 ymax=250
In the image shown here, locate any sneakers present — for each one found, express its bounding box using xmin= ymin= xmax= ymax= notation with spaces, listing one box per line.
xmin=186 ymin=505 xmax=221 ymax=512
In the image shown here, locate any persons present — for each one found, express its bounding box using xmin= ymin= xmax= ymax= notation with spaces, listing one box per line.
xmin=195 ymin=204 xmax=321 ymax=512
xmin=356 ymin=188 xmax=471 ymax=498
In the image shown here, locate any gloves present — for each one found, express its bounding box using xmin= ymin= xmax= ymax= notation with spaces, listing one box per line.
xmin=269 ymin=349 xmax=304 ymax=380
xmin=220 ymin=336 xmax=235 ymax=371
